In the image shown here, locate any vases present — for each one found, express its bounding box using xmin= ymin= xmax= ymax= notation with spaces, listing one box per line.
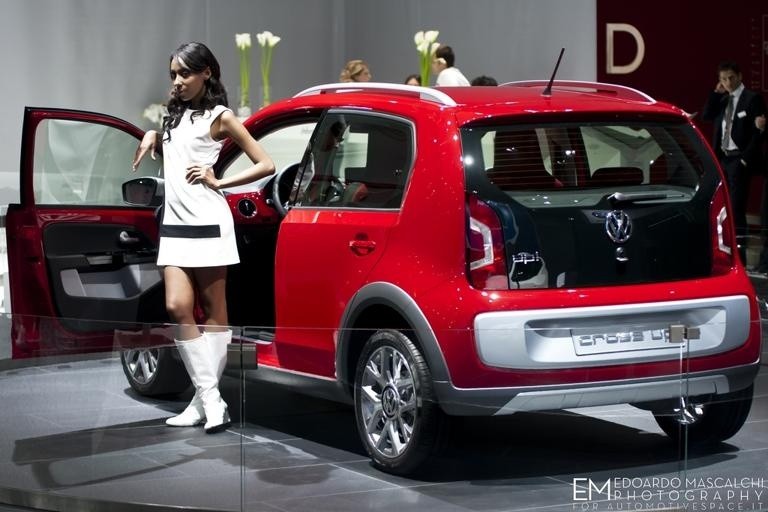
xmin=238 ymin=107 xmax=251 ymax=123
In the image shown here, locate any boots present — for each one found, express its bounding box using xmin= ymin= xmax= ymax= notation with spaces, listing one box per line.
xmin=166 ymin=328 xmax=232 ymax=426
xmin=173 ymin=335 xmax=231 ymax=431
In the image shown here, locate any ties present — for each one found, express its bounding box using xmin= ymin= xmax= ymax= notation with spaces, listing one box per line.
xmin=721 ymin=95 xmax=733 ymax=152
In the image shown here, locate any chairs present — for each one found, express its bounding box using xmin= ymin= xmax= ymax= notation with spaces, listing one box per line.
xmin=487 ymin=125 xmax=564 ymax=190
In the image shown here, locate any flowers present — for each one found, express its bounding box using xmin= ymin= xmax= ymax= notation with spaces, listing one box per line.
xmin=413 ymin=29 xmax=441 ymax=87
xmin=234 ymin=28 xmax=252 ymax=107
xmin=254 ymin=29 xmax=281 ymax=106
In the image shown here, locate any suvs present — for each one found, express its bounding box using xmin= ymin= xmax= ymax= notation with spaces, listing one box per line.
xmin=5 ymin=47 xmax=764 ymax=478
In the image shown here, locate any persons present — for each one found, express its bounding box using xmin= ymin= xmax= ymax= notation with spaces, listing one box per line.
xmin=130 ymin=41 xmax=276 ymax=432
xmin=702 ymin=63 xmax=768 ymax=267
xmin=431 ymin=45 xmax=472 ymax=88
xmin=404 ymin=74 xmax=422 ymax=86
xmin=338 ymin=59 xmax=372 ymax=180
xmin=471 ymin=74 xmax=498 ymax=86
